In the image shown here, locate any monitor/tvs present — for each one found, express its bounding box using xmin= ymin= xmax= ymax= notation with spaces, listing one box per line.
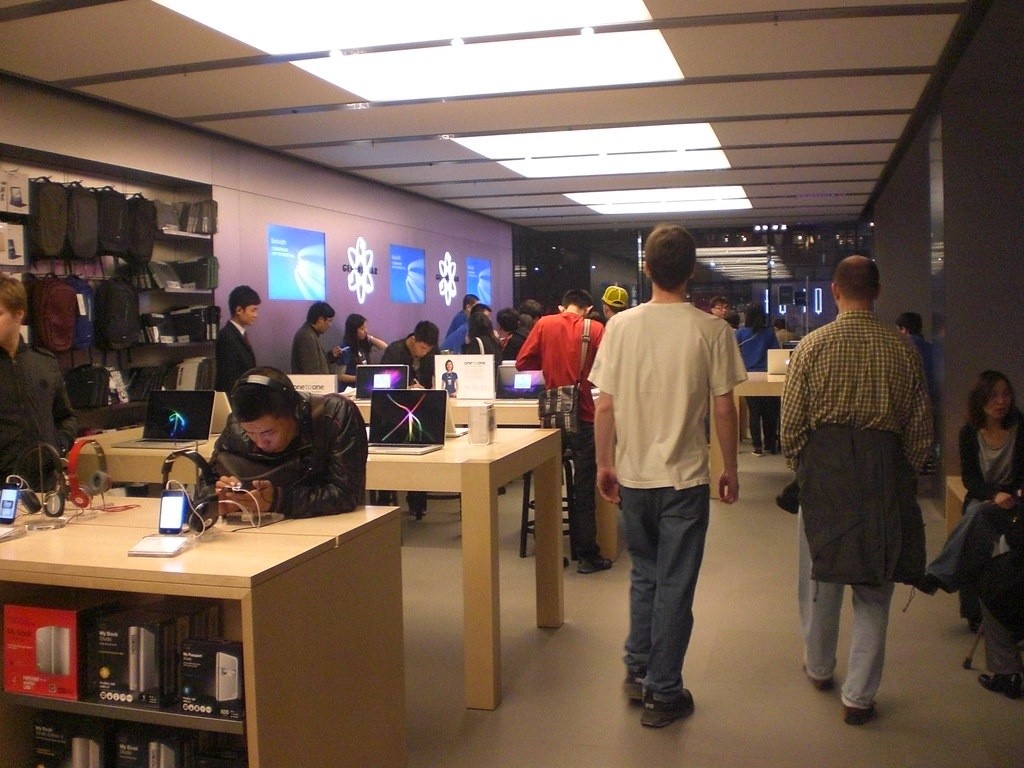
xmin=390 ymin=244 xmax=425 ymax=303
xmin=267 ymin=224 xmax=326 ymax=301
xmin=465 ymin=256 xmax=491 ymax=306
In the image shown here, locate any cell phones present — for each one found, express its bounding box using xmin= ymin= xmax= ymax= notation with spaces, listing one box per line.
xmin=224 ymin=486 xmax=247 ymax=494
xmin=0 ymin=483 xmax=20 ymax=525
xmin=158 ymin=489 xmax=185 ymax=535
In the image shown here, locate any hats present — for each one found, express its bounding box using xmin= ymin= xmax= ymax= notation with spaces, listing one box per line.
xmin=602 ymin=286 xmax=629 ymax=308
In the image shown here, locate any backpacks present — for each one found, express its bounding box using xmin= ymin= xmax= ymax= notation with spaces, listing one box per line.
xmin=30 ymin=176 xmax=157 ymax=410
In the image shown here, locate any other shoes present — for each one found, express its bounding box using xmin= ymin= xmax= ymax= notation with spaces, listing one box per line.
xmin=641 ymin=688 xmax=693 ymax=727
xmin=844 ymin=706 xmax=875 ymax=725
xmin=764 ymin=446 xmax=776 ymax=453
xmin=804 ymin=667 xmax=831 ymax=690
xmin=907 ymin=568 xmax=938 ymax=595
xmin=966 ymin=618 xmax=981 ymax=632
xmin=409 ymin=505 xmax=428 ymax=516
xmin=629 ymin=697 xmax=644 ymax=707
xmin=752 ymin=449 xmax=764 ymax=457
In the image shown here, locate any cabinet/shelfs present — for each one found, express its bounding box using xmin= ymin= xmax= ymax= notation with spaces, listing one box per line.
xmin=1 ymin=143 xmax=219 ymax=433
xmin=0 ymin=489 xmax=406 ymax=767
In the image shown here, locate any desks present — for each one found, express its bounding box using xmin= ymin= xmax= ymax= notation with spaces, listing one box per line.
xmin=77 ymin=362 xmax=790 ymax=710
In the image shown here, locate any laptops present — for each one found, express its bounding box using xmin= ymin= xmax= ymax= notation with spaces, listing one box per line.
xmin=484 ymin=365 xmax=547 ymax=404
xmin=112 ymin=389 xmax=217 ymax=449
xmin=348 ymin=364 xmax=468 ymax=454
xmin=768 ymin=348 xmax=796 ymax=375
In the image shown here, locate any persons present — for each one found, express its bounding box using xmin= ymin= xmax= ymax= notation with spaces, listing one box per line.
xmin=895 ymin=313 xmax=931 ymax=368
xmin=0 ymin=272 xmax=77 ymax=494
xmin=214 ymin=285 xmax=262 ymax=391
xmin=380 ymin=320 xmax=441 ymax=388
xmin=601 ymin=286 xmax=628 ymax=319
xmin=916 ymin=371 xmax=1024 ymax=634
xmin=733 ymin=303 xmax=780 ymax=457
xmin=515 ymin=289 xmax=611 ymax=574
xmin=586 ymin=225 xmax=748 ymax=727
xmin=193 ymin=366 xmax=368 ymax=518
xmin=709 ymin=296 xmax=729 ymax=318
xmin=773 ymin=318 xmax=799 ymax=348
xmin=335 ymin=313 xmax=388 ymax=393
xmin=291 ymin=302 xmax=342 ymax=374
xmin=976 ymin=550 xmax=1024 ymax=700
xmin=439 ymin=294 xmax=543 ymax=377
xmin=780 ymin=255 xmax=934 ymax=724
xmin=441 ymin=360 xmax=458 ymax=398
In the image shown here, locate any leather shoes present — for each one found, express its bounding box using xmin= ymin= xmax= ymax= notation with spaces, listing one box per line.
xmin=979 ymin=671 xmax=1021 ymax=700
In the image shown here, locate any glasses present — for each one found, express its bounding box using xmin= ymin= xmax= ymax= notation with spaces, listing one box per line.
xmin=325 ymin=318 xmax=333 ymax=323
xmin=714 ymin=306 xmax=728 ymax=311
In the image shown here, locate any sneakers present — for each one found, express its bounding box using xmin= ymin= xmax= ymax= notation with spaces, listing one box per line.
xmin=563 ymin=557 xmax=569 ymax=569
xmin=577 ymin=556 xmax=612 ymax=573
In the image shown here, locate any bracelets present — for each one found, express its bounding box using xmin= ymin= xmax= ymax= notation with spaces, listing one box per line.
xmin=370 ymin=336 xmax=373 ymax=338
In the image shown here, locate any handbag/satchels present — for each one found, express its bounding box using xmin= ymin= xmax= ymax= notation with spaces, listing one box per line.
xmin=538 ymin=383 xmax=579 ymax=434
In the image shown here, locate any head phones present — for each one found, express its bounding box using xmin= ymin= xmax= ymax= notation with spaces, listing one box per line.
xmin=162 ymin=449 xmax=218 ymax=533
xmin=230 ymin=376 xmax=311 ymax=425
xmin=68 ymin=439 xmax=113 ymax=508
xmin=13 ymin=442 xmax=66 ymax=517
